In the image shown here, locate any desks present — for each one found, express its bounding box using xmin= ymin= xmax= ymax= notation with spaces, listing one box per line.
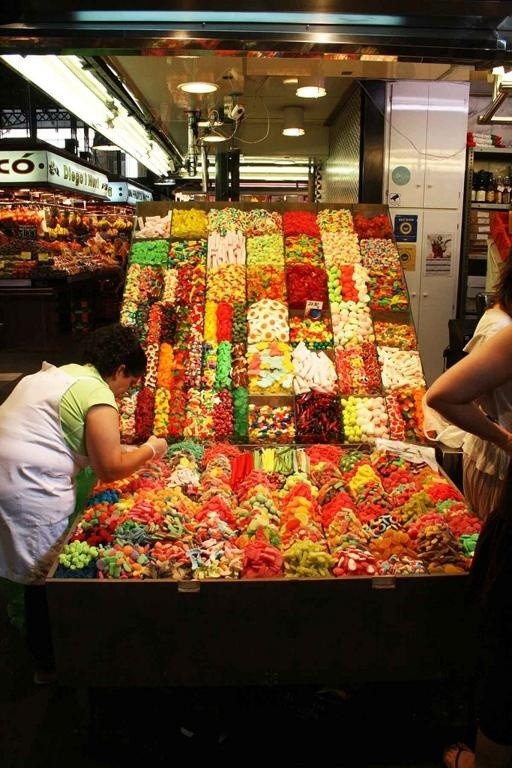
xmin=0 ymin=267 xmax=127 ymax=353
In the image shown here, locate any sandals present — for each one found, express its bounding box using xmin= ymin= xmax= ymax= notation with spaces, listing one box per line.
xmin=444 ymin=740 xmax=476 ymax=768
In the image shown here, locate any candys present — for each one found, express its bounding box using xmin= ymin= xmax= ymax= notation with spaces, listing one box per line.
xmin=114 ymin=208 xmax=439 ymax=447
xmin=57 ymin=438 xmax=481 ymax=580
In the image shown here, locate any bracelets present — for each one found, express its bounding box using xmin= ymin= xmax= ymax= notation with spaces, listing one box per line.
xmin=141 ymin=441 xmax=157 ymax=463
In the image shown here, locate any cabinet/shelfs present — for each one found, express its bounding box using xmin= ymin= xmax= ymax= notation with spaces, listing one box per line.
xmin=460 ymin=147 xmax=512 ymax=319
xmin=359 ymin=79 xmax=472 ymax=392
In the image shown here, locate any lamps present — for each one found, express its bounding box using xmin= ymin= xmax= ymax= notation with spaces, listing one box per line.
xmin=481 ymin=61 xmax=511 ymax=123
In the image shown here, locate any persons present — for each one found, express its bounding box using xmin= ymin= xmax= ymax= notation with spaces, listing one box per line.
xmin=0 ymin=323 xmax=169 ymax=691
xmin=458 ymin=250 xmax=510 ymax=522
xmin=425 ymin=317 xmax=510 ymax=767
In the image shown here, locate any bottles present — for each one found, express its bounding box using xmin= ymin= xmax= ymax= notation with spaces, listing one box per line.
xmin=471 ymin=176 xmax=512 ymax=204
